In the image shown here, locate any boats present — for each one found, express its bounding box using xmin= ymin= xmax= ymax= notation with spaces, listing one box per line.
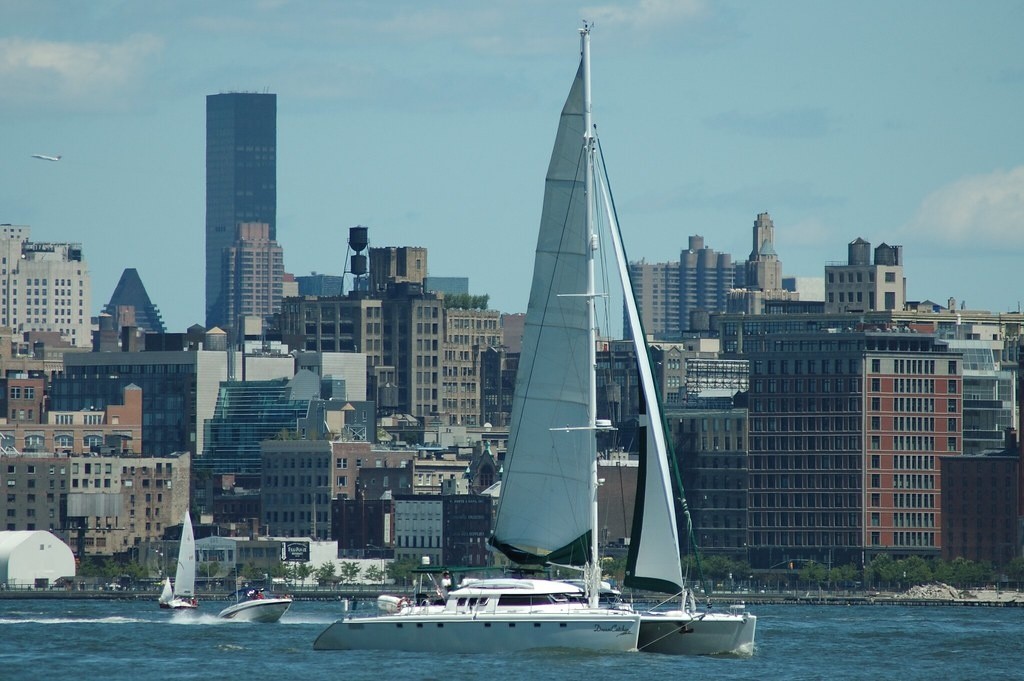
xmin=218 ymin=588 xmax=292 ymax=622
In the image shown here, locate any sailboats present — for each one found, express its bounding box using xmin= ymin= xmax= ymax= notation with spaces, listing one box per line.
xmin=313 ymin=21 xmax=758 ymax=652
xmin=158 ymin=508 xmax=198 ymax=612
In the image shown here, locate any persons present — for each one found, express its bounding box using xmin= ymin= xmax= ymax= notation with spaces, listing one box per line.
xmin=441 ymin=572 xmax=451 ymax=590
xmin=2 ymin=582 xmax=7 ymax=591
xmin=105 ymin=582 xmax=109 ymax=590
xmin=260 ymin=590 xmax=266 ymax=600
xmin=686 ymin=593 xmax=693 ymax=607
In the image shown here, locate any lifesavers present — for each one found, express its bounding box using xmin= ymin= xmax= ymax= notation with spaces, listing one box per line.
xmin=396 ymin=598 xmax=411 ymax=613
